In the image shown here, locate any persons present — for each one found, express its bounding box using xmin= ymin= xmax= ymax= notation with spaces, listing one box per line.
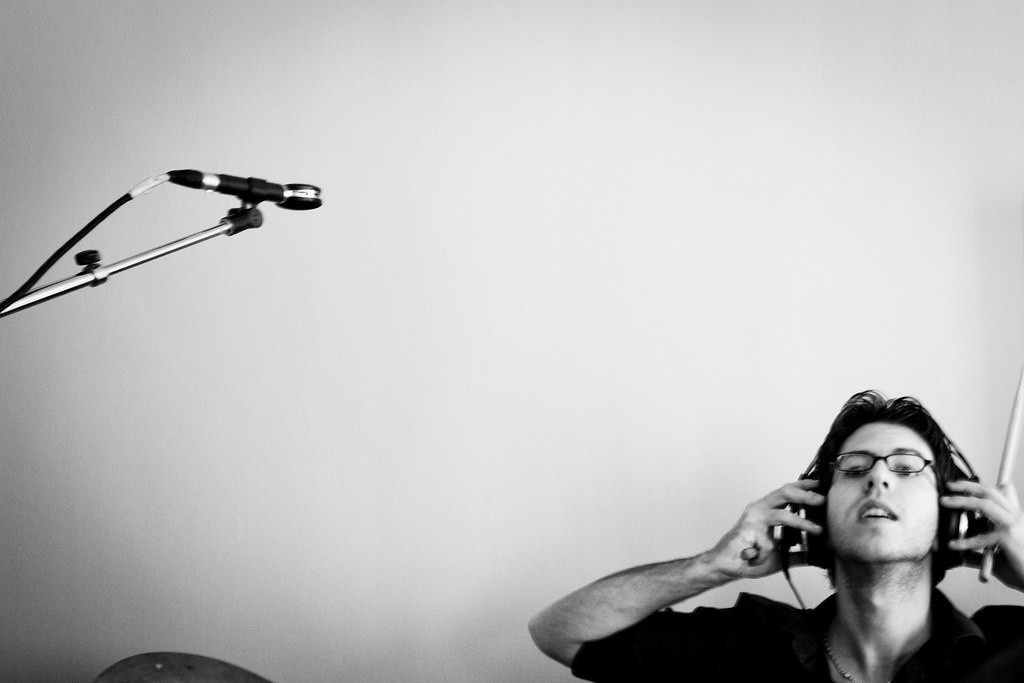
xmin=529 ymin=390 xmax=1024 ymax=683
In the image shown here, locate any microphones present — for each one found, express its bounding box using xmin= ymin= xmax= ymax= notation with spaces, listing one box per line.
xmin=166 ymin=169 xmax=323 ymax=210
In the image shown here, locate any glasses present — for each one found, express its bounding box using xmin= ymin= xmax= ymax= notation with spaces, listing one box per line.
xmin=829 ymin=451 xmax=940 ymax=489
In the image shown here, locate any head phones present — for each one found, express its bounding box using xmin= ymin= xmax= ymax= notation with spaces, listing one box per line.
xmin=779 ymin=436 xmax=986 ymax=569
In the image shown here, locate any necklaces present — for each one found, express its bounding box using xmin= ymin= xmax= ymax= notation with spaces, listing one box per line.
xmin=825 ymin=642 xmax=861 ymax=683
